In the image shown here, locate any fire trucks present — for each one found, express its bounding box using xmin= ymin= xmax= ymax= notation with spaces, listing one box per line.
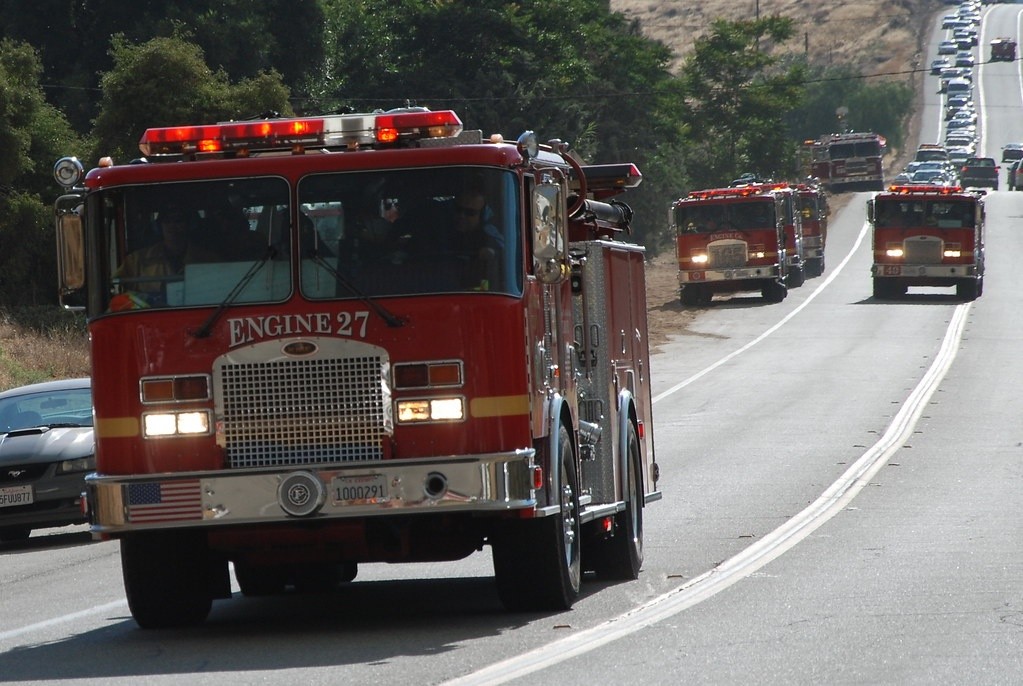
xmin=806 ymin=129 xmax=887 ymax=193
xmin=670 ymin=171 xmax=832 ymax=306
xmin=55 ymin=106 xmax=666 ymax=632
xmin=867 ymin=177 xmax=987 ymax=302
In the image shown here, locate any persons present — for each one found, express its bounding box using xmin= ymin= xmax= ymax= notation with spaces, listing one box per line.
xmin=447 ymin=188 xmax=496 ymax=261
xmin=113 ymin=198 xmax=224 ymax=292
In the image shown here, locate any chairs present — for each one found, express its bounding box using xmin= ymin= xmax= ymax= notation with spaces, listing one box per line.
xmin=9 ymin=411 xmax=43 ymax=428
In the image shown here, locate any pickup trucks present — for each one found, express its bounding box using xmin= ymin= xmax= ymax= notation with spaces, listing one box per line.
xmin=955 ymin=157 xmax=1002 ymax=192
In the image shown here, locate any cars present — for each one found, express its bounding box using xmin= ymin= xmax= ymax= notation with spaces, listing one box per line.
xmin=1000 ymin=143 xmax=1023 ymax=163
xmin=898 ymin=0 xmax=1017 ymax=187
xmin=1012 ymin=157 xmax=1023 ymax=191
xmin=0 ymin=378 xmax=97 ymax=547
xmin=1006 ymin=160 xmax=1020 ymax=190
